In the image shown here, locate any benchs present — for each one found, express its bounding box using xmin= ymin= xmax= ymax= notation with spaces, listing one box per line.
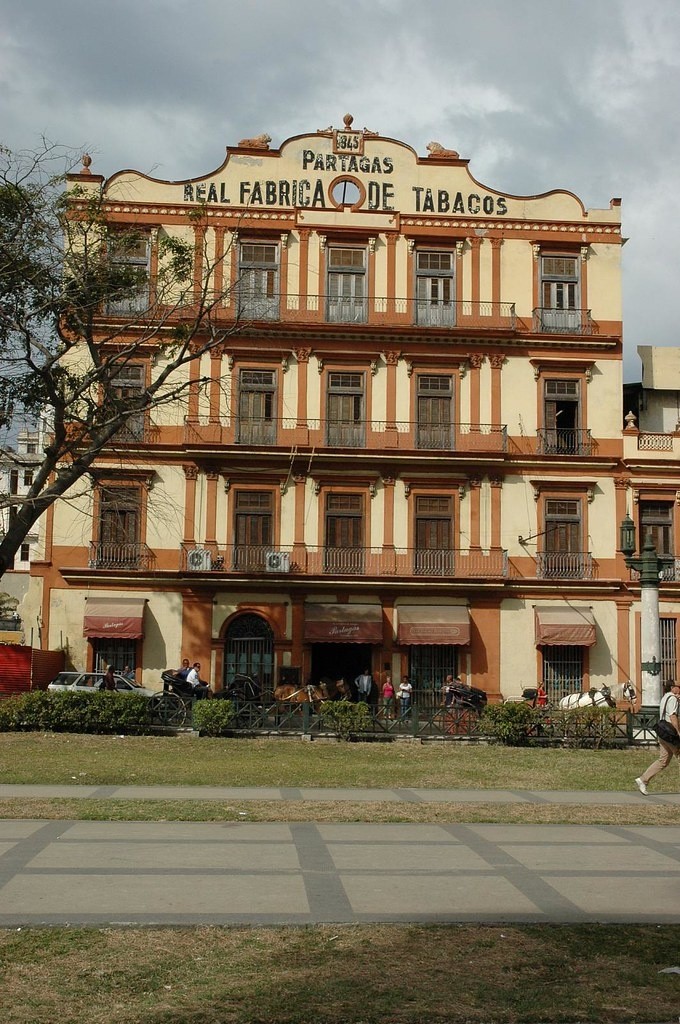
xmin=162 ymin=669 xmax=208 ymax=696
xmin=453 ymin=680 xmax=487 ymax=708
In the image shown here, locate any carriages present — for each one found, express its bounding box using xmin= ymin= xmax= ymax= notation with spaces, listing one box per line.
xmin=159 ymin=669 xmax=352 ymax=729
xmin=439 ymin=675 xmax=638 ymax=734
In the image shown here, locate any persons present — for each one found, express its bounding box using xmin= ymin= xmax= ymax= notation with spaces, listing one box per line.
xmin=442 ymin=675 xmax=462 ymax=705
xmin=399 ymin=675 xmax=413 ymax=721
xmin=354 ymin=667 xmax=372 ymax=702
xmin=537 ymin=681 xmax=549 ymax=711
xmin=103 ymin=664 xmax=116 ymax=689
xmin=173 ymin=659 xmax=208 ymax=698
xmin=383 ymin=676 xmax=394 ymax=719
xmin=122 ymin=665 xmax=135 ymax=679
xmin=634 ymin=679 xmax=680 ymax=797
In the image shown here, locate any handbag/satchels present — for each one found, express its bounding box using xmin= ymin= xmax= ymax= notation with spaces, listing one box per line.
xmin=395 ymin=691 xmax=403 ymax=697
xmin=652 ymin=720 xmax=680 ymax=745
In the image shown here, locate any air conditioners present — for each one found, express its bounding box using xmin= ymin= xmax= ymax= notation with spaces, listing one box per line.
xmin=266 ymin=553 xmax=290 ymax=573
xmin=186 ymin=550 xmax=211 ymax=571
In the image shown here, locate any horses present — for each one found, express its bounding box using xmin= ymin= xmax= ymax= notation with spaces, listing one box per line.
xmin=558 ymin=680 xmax=637 ymax=740
xmin=272 ymin=678 xmax=352 ymax=718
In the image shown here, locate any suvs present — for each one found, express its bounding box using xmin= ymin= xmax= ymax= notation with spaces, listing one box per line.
xmin=45 ymin=672 xmax=165 ymax=709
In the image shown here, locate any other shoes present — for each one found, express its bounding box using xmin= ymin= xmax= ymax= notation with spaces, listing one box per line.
xmin=634 ymin=778 xmax=648 ymax=796
xmin=388 ymin=715 xmax=391 ymax=719
xmin=383 ymin=715 xmax=387 ymax=718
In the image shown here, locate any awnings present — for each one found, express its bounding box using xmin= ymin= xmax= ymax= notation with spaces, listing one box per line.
xmin=533 ymin=606 xmax=597 ymax=648
xmin=397 ymin=606 xmax=472 ymax=646
xmin=83 ymin=597 xmax=149 ymax=639
xmin=304 ymin=605 xmax=383 ymax=644
